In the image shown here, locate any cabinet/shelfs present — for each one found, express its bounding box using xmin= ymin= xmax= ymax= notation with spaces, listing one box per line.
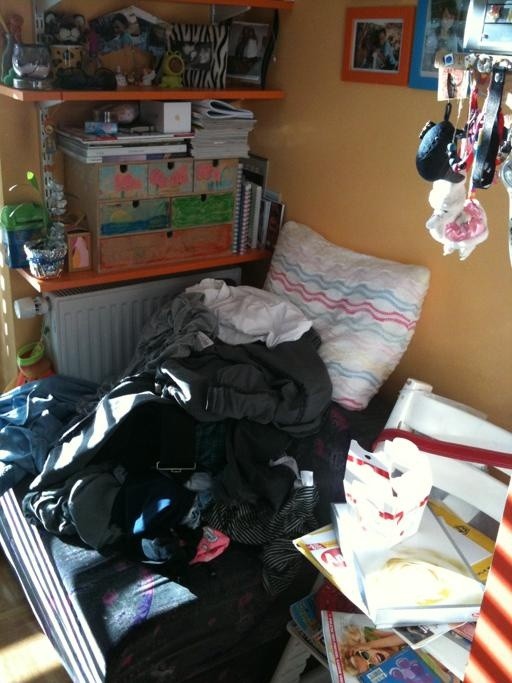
xmin=1 ymin=85 xmax=285 ymax=289
xmin=65 ymin=154 xmax=240 ymax=274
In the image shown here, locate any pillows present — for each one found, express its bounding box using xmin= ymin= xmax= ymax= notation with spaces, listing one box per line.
xmin=263 ymin=220 xmax=431 ymax=413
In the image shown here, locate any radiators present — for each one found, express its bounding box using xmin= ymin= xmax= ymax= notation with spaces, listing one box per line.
xmin=13 ymin=267 xmax=254 ymax=387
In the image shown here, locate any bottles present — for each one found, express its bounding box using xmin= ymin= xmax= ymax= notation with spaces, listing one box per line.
xmin=1 ymin=33 xmax=15 ymax=84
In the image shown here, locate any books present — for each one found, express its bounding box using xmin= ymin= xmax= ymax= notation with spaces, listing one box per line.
xmin=54 ymin=124 xmax=194 ymax=164
xmin=188 ymin=99 xmax=255 ymax=159
xmin=360 ymin=648 xmax=444 ymax=683
xmin=321 ymin=610 xmax=402 ymax=683
xmin=233 ymin=154 xmax=286 ymax=254
xmin=424 ymin=621 xmax=477 ymax=682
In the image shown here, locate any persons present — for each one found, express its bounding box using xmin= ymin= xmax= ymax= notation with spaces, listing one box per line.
xmin=337 ymin=624 xmax=406 ymax=678
xmin=425 ymin=1 xmax=464 ymax=72
xmin=359 ymin=33 xmax=388 ymax=71
xmin=376 ymin=28 xmax=397 ymax=68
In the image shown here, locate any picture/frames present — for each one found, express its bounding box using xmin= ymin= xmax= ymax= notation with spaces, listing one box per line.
xmin=340 ymin=7 xmax=418 ymax=86
xmin=409 ymin=0 xmax=470 ymax=90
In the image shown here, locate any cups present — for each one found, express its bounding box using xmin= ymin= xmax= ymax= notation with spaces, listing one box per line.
xmin=50 ymin=43 xmax=84 ymax=82
xmin=93 ymin=109 xmax=116 ymax=137
